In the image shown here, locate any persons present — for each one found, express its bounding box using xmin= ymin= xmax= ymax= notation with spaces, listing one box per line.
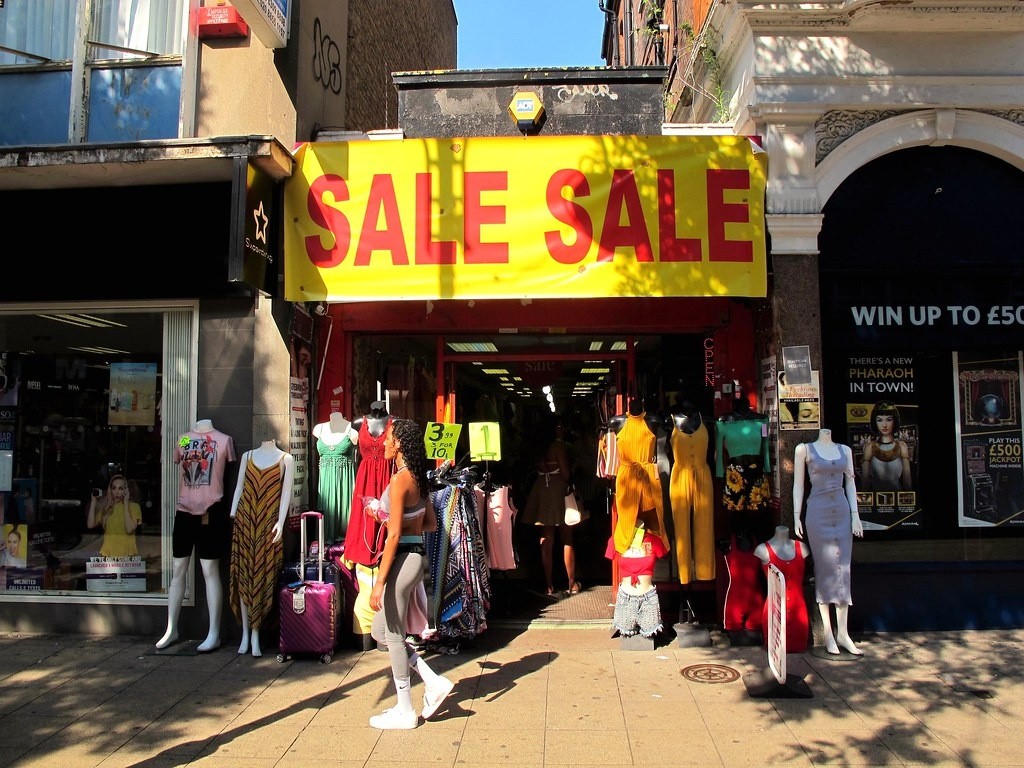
xmin=86 ymin=476 xmax=143 ymax=558
xmin=604 ymin=519 xmax=667 ymax=637
xmin=228 ymin=438 xmax=295 ymax=657
xmin=343 ymin=401 xmax=401 ymax=565
xmin=0 ymin=530 xmax=26 ymax=568
xmin=312 ymin=411 xmax=359 ymax=546
xmin=608 ymin=396 xmax=772 ymax=585
xmin=754 ymin=526 xmax=811 ymax=654
xmin=792 ymin=428 xmax=866 ymax=657
xmin=155 ymin=419 xmax=236 ymax=651
xmin=520 ymin=430 xmax=583 ymax=599
xmin=369 ymin=420 xmax=456 ymax=730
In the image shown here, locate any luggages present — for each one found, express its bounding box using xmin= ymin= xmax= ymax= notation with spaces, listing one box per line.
xmin=277 ymin=512 xmax=336 ymax=665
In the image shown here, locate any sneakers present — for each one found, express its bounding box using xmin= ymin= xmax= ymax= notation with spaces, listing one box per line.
xmin=422 ymin=675 xmax=454 ymax=719
xmin=369 ymin=707 xmax=418 ymax=730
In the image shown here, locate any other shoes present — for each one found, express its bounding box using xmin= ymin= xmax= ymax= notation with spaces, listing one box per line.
xmin=569 ymin=580 xmax=581 ymax=594
xmin=546 ymin=585 xmax=554 ymax=595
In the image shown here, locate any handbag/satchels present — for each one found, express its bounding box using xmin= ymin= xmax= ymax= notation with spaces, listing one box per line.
xmin=564 ymin=484 xmax=589 ymax=526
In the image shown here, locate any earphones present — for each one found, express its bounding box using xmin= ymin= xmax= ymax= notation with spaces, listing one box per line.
xmin=396 ymin=442 xmax=399 ymax=446
